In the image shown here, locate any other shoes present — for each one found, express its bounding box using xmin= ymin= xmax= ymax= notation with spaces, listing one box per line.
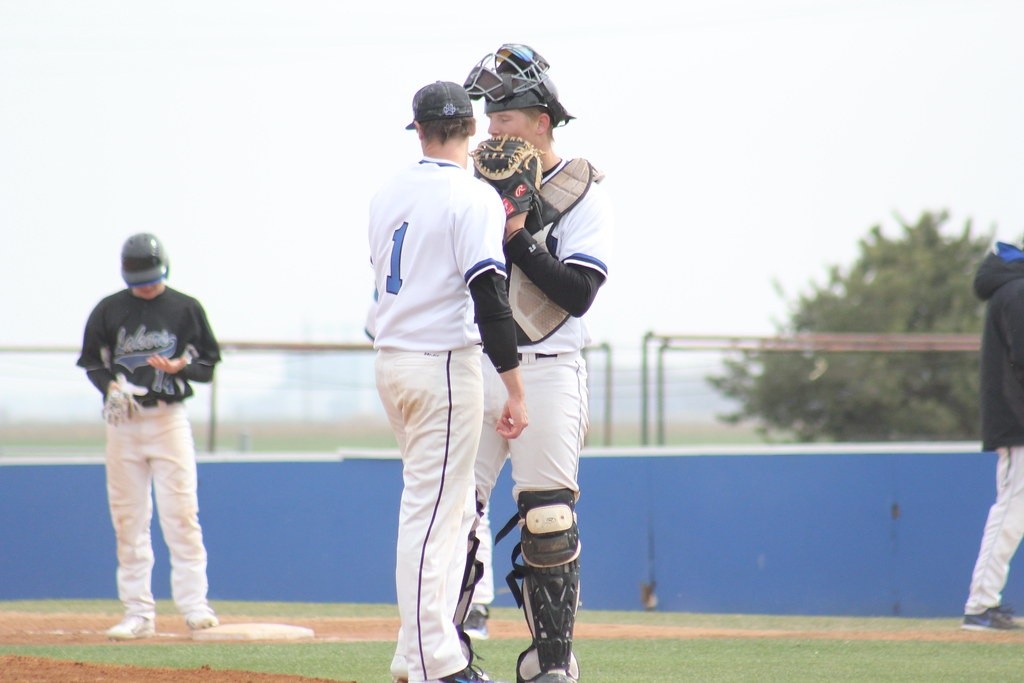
xmin=538 ymin=672 xmax=577 ymax=683
xmin=439 ymin=664 xmax=494 ymax=683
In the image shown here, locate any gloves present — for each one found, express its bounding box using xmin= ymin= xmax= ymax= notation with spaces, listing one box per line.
xmin=104 ymin=390 xmax=142 ymax=426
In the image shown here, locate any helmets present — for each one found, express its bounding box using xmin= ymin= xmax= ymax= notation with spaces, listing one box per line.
xmin=483 ymin=72 xmax=576 ymax=128
xmin=122 ymin=234 xmax=167 ymax=285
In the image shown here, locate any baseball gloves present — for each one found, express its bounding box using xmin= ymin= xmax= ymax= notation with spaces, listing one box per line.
xmin=473 ymin=136 xmax=544 ymax=233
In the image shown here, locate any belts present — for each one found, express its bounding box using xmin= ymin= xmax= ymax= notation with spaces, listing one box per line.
xmin=482 ymin=348 xmax=559 ymax=359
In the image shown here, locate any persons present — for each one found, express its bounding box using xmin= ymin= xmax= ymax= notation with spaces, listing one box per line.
xmin=364 ymin=82 xmax=529 ymax=683
xmin=960 ymin=228 xmax=1024 ymax=634
xmin=77 ymin=233 xmax=223 ymax=640
xmin=462 ymin=44 xmax=610 ymax=683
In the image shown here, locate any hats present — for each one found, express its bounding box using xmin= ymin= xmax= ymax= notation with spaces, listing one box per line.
xmin=406 ymin=81 xmax=473 ymax=129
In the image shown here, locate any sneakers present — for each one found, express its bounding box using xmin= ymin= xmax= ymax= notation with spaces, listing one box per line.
xmin=186 ymin=607 xmax=218 ymax=630
xmin=963 ymin=604 xmax=1023 ymax=632
xmin=108 ymin=614 xmax=155 ymax=639
